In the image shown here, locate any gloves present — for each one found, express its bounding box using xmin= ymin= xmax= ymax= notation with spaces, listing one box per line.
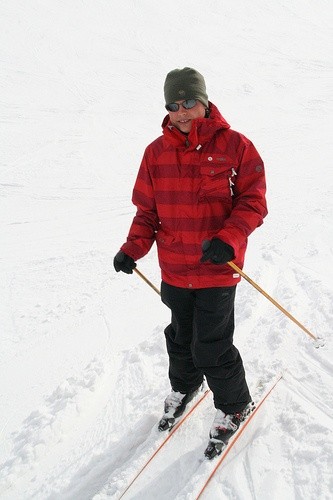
xmin=198 ymin=236 xmax=235 ymax=266
xmin=114 ymin=252 xmax=136 ymax=274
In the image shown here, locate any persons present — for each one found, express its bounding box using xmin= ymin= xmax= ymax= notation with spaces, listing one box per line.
xmin=111 ymin=66 xmax=268 ymax=458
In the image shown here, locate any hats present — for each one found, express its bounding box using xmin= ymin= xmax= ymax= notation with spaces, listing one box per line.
xmin=164 ymin=66 xmax=209 ymax=106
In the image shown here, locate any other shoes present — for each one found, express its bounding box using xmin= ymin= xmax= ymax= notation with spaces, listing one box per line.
xmin=204 ymin=403 xmax=254 ymax=437
xmin=164 ymin=387 xmax=200 ymax=416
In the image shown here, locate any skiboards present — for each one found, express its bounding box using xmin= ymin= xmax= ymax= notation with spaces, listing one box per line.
xmin=87 ymin=352 xmax=281 ymax=500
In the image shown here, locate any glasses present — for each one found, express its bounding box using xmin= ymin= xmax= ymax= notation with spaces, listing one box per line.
xmin=165 ymin=99 xmax=204 ymax=111
